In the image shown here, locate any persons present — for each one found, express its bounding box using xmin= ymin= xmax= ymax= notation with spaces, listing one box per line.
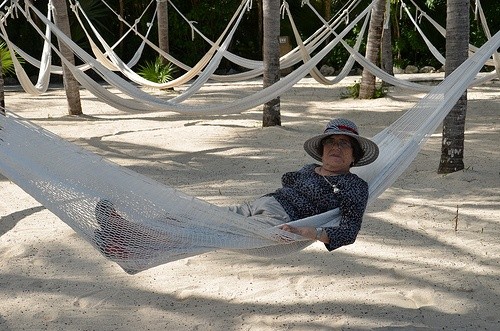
xmin=93 ymin=119 xmax=379 ymax=275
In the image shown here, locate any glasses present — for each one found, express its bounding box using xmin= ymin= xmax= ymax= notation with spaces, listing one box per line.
xmin=321 ymin=139 xmax=352 ymax=149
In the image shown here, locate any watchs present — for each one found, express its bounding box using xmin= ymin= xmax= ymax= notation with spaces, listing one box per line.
xmin=315 ymin=226 xmax=324 ymax=240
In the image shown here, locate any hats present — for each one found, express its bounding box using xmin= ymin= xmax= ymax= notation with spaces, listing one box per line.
xmin=303 ymin=118 xmax=379 ymax=167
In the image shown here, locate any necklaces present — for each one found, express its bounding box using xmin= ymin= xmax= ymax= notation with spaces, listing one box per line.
xmin=320 ymin=167 xmax=350 ymax=193
